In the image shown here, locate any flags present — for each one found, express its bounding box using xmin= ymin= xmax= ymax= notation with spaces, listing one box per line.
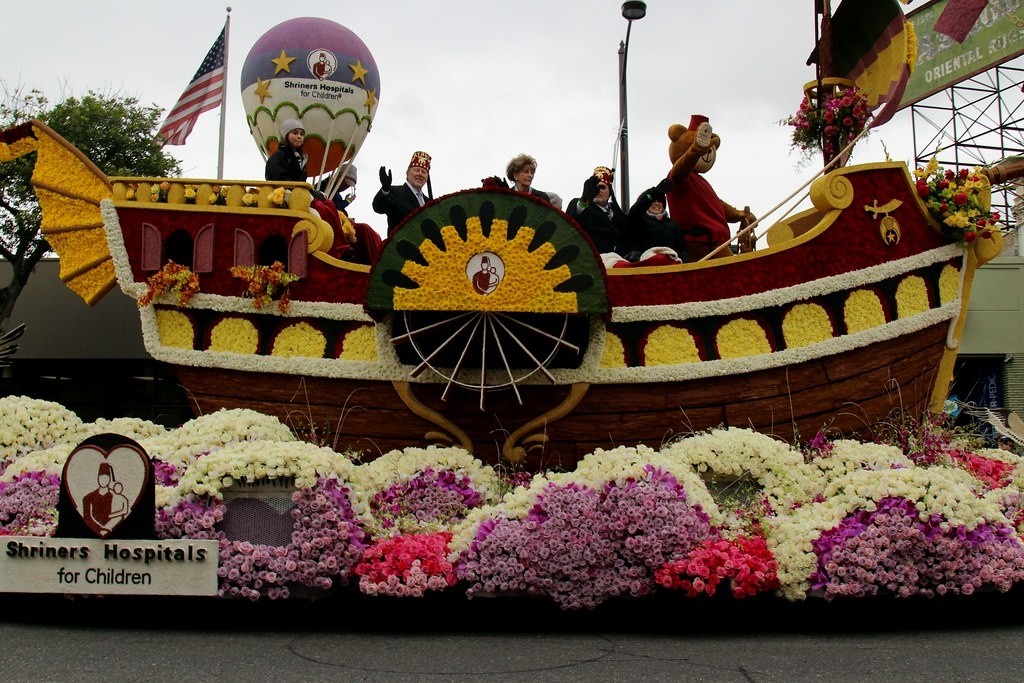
xmin=151 ymin=21 xmax=226 ymax=148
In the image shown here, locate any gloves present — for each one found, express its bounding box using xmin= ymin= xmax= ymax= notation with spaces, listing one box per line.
xmin=379 ymin=166 xmax=392 ymax=192
xmin=580 ymin=176 xmax=601 ymax=205
xmin=656 ymin=179 xmax=675 ymax=194
xmin=494 ymin=176 xmax=509 ymax=188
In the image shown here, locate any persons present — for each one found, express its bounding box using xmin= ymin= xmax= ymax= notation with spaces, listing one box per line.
xmin=372 ymin=151 xmax=434 ymax=238
xmin=566 ymin=166 xmax=690 ymax=263
xmin=506 ymin=155 xmax=550 ymax=204
xmin=265 ymin=119 xmax=328 ymax=201
xmin=314 ymin=164 xmax=357 ymax=217
xmin=996 ymin=434 xmax=1014 ymax=453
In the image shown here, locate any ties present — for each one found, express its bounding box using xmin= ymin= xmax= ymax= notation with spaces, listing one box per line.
xmin=417 ymin=193 xmax=423 ymax=206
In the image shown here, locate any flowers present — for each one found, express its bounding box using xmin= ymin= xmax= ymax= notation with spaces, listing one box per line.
xmin=0 ymin=372 xmax=1024 ymax=611
xmin=137 ymin=257 xmax=203 ymax=312
xmin=771 ymin=85 xmax=878 ymax=172
xmin=880 ymin=134 xmax=1019 ymax=243
xmin=121 ymin=180 xmax=294 ymax=207
xmin=230 ymin=256 xmax=299 ymax=314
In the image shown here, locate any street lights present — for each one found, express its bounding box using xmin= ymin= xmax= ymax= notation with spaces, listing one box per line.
xmin=617 ymin=0 xmax=648 ymax=215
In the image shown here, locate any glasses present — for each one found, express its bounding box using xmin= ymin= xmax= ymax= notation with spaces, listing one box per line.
xmin=994 ymin=438 xmax=1011 ymax=445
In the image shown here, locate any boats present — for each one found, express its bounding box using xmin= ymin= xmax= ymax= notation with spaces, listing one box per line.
xmin=25 ymin=0 xmax=1012 ymax=475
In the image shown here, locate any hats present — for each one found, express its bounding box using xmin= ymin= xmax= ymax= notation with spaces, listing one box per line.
xmin=593 ymin=166 xmax=620 ymax=210
xmin=409 ymin=152 xmax=433 ymax=200
xmin=339 ymin=164 xmax=357 ymax=185
xmin=284 ymin=119 xmax=305 ymax=138
xmin=637 ymin=187 xmax=666 ymax=210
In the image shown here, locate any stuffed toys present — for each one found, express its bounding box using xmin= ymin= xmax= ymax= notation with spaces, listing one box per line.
xmin=666 ymin=114 xmax=757 ymax=263
